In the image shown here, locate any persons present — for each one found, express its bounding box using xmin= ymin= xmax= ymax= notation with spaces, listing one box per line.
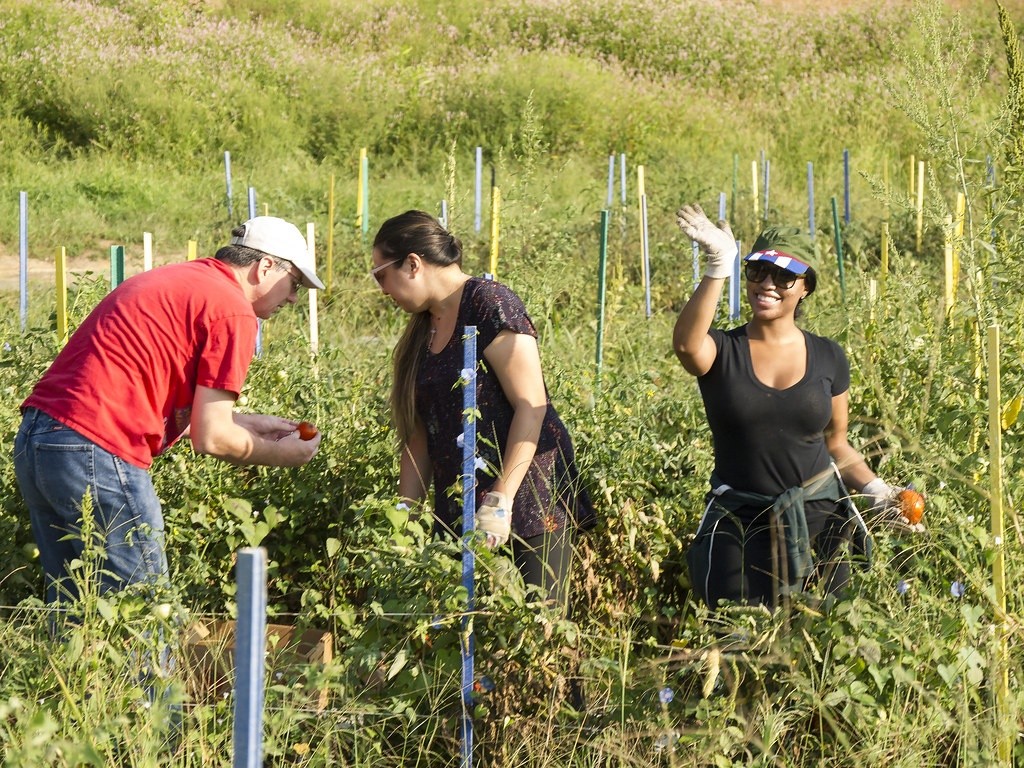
xmin=372 ymin=210 xmax=598 ymax=619
xmin=13 ymin=217 xmax=326 ymax=754
xmin=672 ymin=202 xmax=926 ymax=605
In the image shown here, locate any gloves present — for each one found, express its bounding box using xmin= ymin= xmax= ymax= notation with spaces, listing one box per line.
xmin=863 ymin=484 xmax=926 ymax=534
xmin=676 ymin=202 xmax=738 ymax=279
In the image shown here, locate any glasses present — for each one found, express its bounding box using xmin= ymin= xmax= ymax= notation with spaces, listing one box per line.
xmin=745 ymin=263 xmax=808 ymax=290
xmin=371 ymin=253 xmax=426 ymax=287
xmin=255 ymin=255 xmax=303 ymax=293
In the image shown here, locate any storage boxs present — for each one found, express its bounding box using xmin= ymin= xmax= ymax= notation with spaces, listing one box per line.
xmin=178 ymin=618 xmax=334 ymax=715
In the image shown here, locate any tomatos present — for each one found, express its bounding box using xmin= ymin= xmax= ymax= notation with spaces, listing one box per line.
xmin=296 ymin=422 xmax=318 ymax=440
xmin=892 ymin=489 xmax=925 ymax=525
xmin=236 ymin=393 xmax=248 ymax=405
xmin=276 ymin=371 xmax=286 ymax=380
xmin=23 ymin=543 xmax=40 ymax=562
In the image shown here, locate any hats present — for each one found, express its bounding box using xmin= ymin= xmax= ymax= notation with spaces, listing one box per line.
xmin=744 ymin=225 xmax=817 ymax=297
xmin=231 ymin=215 xmax=326 ymax=291
